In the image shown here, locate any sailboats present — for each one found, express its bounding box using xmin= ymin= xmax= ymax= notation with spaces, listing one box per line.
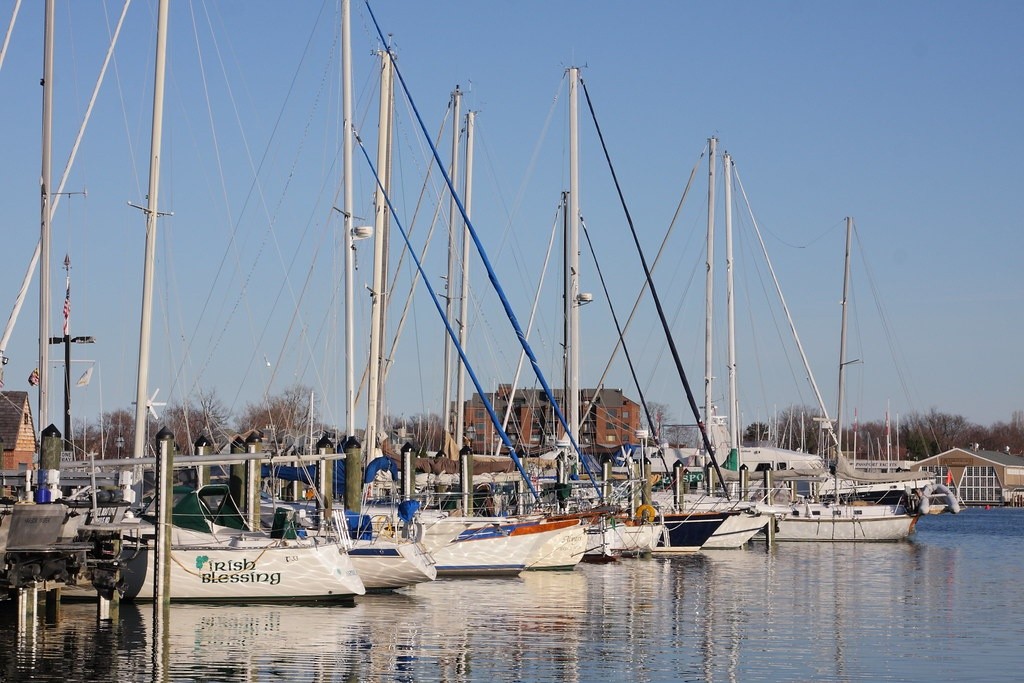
xmin=0 ymin=0 xmax=970 ymax=604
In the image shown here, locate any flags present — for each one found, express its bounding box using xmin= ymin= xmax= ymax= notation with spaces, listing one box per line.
xmin=62 ymin=281 xmax=72 ymax=333
xmin=76 ymin=367 xmax=93 ymax=386
xmin=27 ymin=367 xmax=40 ymax=386
